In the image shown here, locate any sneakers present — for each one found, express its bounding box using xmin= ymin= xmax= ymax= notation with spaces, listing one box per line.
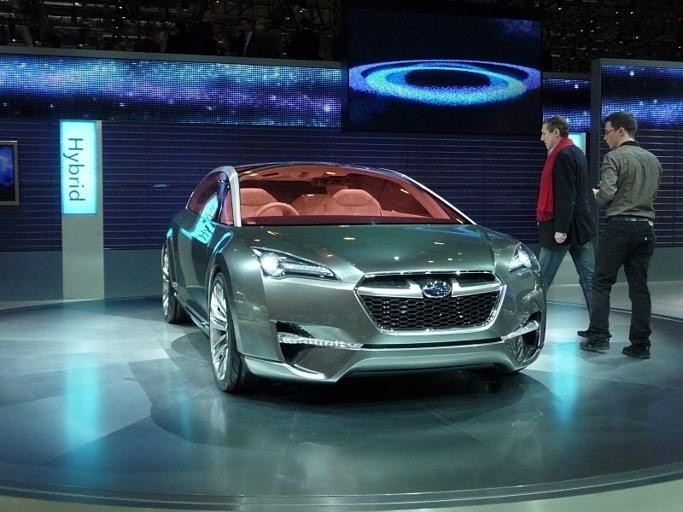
xmin=623 ymin=343 xmax=651 ymax=358
xmin=580 ymin=335 xmax=609 ymax=350
xmin=577 ymin=329 xmax=612 ymax=338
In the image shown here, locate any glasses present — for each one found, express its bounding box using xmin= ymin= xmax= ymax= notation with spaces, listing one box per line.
xmin=604 ymin=127 xmax=618 ymax=135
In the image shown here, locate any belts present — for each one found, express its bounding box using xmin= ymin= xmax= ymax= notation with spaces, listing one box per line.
xmin=611 ymin=216 xmax=648 ymax=222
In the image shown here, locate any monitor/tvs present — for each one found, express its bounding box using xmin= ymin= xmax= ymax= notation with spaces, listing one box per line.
xmin=0 ymin=141 xmax=21 ymax=206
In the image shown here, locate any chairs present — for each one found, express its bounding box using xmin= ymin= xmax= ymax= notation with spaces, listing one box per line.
xmin=219 ymin=188 xmax=383 ymax=225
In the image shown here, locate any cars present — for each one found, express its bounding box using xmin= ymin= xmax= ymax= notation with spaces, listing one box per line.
xmin=157 ymin=157 xmax=551 ymax=399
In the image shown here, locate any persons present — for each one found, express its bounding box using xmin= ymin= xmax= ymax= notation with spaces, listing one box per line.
xmin=593 ymin=18 xmax=637 ymax=60
xmin=187 ymin=3 xmax=231 ymax=56
xmin=66 ymin=14 xmax=98 ymax=51
xmin=211 ymin=21 xmax=226 ymax=46
xmin=533 ymin=114 xmax=599 ymax=339
xmin=219 ymin=16 xmax=254 ymax=56
xmin=13 ymin=0 xmax=57 ymax=47
xmin=283 ymin=16 xmax=325 ymax=60
xmin=578 ymin=109 xmax=663 ymax=361
xmin=245 ymin=16 xmax=277 ymax=56
xmin=562 ymin=14 xmax=602 ymax=73
